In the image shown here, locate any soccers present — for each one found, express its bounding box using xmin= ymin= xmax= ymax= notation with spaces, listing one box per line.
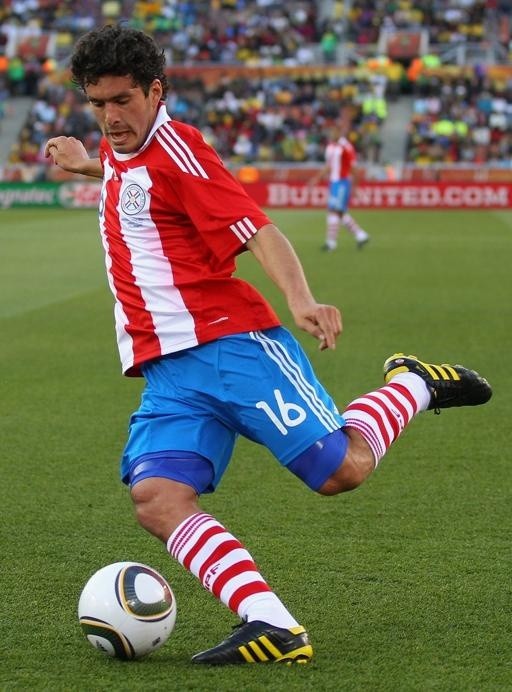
xmin=79 ymin=562 xmax=176 ymax=660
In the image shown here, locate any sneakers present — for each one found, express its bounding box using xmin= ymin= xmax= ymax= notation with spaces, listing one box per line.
xmin=320 ymin=240 xmax=338 ymax=250
xmin=355 ymin=234 xmax=369 ymax=250
xmin=191 ymin=619 xmax=315 ymax=668
xmin=384 ymin=352 xmax=494 ymax=413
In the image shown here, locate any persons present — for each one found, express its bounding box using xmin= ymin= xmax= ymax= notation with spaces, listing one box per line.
xmin=43 ymin=23 xmax=494 ymax=667
xmin=309 ymin=124 xmax=371 ymax=251
xmin=1 ymin=1 xmax=512 ymax=181
